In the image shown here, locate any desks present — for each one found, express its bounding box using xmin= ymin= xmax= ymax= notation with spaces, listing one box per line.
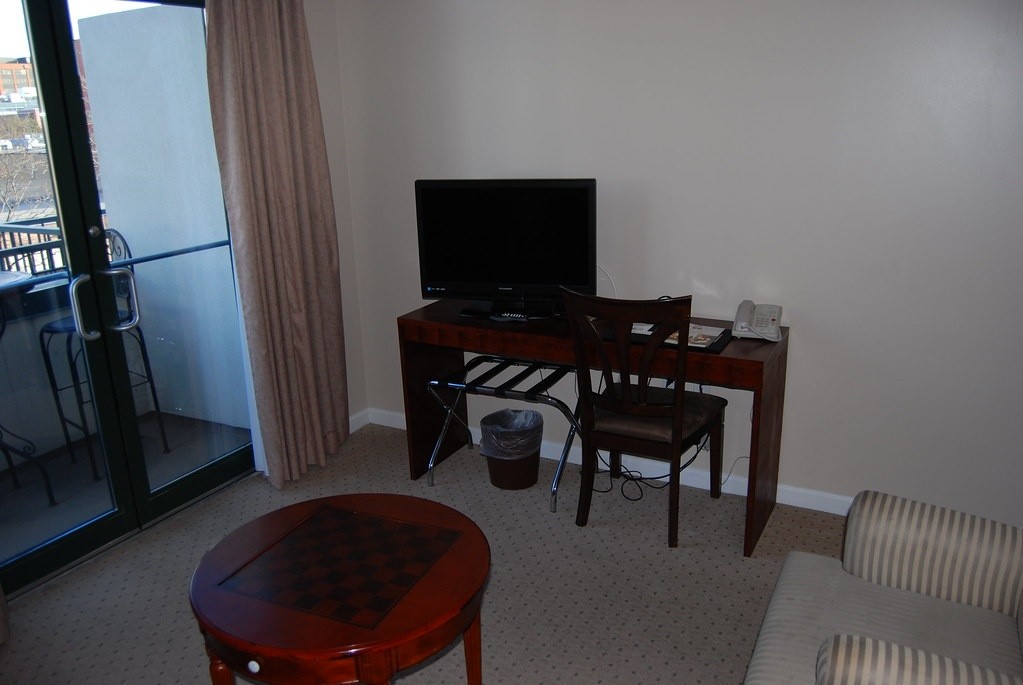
xmin=0 ymin=271 xmax=58 ymax=509
xmin=396 ymin=299 xmax=790 ymax=557
xmin=189 ymin=492 xmax=491 ymax=685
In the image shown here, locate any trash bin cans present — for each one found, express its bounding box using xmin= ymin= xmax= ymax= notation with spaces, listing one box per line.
xmin=480 ymin=407 xmax=545 ymax=490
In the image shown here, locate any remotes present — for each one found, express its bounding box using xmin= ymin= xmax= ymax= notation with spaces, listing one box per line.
xmin=490 ymin=313 xmax=529 ymax=322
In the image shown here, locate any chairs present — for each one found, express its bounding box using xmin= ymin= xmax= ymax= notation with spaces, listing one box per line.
xmin=39 ymin=228 xmax=170 ymax=484
xmin=558 ymin=285 xmax=728 ymax=550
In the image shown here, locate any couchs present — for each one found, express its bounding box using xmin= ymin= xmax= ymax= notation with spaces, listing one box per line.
xmin=743 ymin=490 xmax=1023 ymax=685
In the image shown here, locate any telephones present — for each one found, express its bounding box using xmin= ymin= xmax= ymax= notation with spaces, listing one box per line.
xmin=733 ymin=299 xmax=782 ymax=341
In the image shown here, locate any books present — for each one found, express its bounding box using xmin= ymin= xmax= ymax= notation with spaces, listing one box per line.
xmin=665 ymin=322 xmax=725 ymax=348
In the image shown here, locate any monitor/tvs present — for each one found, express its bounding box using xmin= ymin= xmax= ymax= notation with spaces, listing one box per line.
xmin=413 ymin=178 xmax=597 ymax=320
